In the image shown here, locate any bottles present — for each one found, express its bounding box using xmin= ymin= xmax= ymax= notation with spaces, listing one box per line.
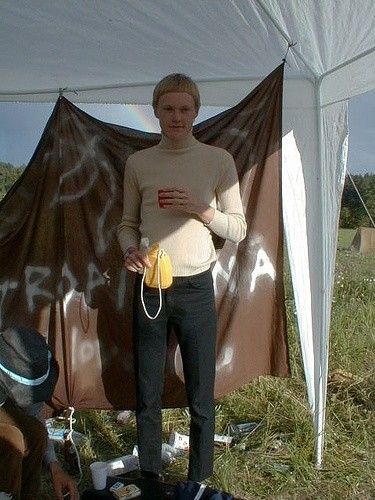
xmin=138 ymin=237 xmax=149 ymax=274
xmin=116 ymin=410 xmax=132 ymax=425
xmin=64 ymin=433 xmax=80 ymax=476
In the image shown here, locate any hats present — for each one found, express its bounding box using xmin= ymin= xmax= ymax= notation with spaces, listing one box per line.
xmin=1 ymin=327 xmax=60 ymax=409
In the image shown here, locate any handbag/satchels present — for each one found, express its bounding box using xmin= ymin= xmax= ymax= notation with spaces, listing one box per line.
xmin=141 ymin=251 xmax=172 ymax=320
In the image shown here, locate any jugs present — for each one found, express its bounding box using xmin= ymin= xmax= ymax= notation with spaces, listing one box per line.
xmin=144 ymin=243 xmax=172 ymax=289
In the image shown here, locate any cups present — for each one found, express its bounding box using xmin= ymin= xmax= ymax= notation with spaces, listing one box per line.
xmin=169 ymin=431 xmax=189 ymax=449
xmin=90 ymin=461 xmax=108 ymax=489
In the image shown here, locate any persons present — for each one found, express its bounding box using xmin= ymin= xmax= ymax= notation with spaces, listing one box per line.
xmin=117 ymin=74 xmax=248 ymax=500
